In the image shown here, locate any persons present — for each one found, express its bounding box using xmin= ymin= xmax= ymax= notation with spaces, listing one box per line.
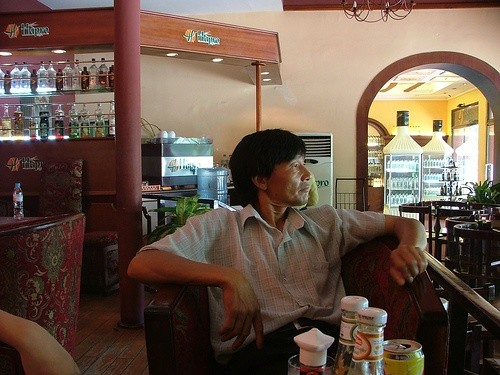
xmin=0 ymin=310 xmax=81 ymax=375
xmin=126 ymin=129 xmax=428 ymax=375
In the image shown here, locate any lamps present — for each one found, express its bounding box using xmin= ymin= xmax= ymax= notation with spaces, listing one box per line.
xmin=342 ymin=0 xmax=416 ymax=23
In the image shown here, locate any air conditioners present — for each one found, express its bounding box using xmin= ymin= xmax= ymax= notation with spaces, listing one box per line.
xmin=292 ymin=133 xmax=333 ymax=207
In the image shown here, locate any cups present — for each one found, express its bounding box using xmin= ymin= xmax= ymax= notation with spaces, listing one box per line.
xmin=161 ymin=131 xmax=168 ymax=144
xmin=168 ymin=131 xmax=175 ymax=142
xmin=287 ymin=354 xmax=335 ymax=375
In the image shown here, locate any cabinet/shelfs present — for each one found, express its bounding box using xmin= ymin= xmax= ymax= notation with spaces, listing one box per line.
xmin=383 ymin=155 xmax=466 ymax=220
xmin=0 ymin=48 xmax=115 ymax=129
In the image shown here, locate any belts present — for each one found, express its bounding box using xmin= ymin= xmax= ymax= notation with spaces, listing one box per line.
xmin=277 ymin=317 xmax=341 ymax=334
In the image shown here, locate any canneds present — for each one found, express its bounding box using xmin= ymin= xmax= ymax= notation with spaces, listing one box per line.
xmin=382 ymin=339 xmax=424 ymax=375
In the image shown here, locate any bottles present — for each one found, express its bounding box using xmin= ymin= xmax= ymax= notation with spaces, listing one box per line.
xmin=332 ymin=295 xmax=369 ymax=375
xmin=0 ymin=57 xmax=115 ymax=95
xmin=13 ymin=183 xmax=24 ymax=220
xmin=294 ymin=328 xmax=335 ymax=375
xmin=387 ymin=159 xmax=462 ymax=205
xmin=347 ymin=307 xmax=388 ymax=375
xmin=0 ymin=100 xmax=116 ymax=142
xmin=197 ymin=168 xmax=228 ymax=203
xmin=368 ymin=135 xmax=385 ymax=188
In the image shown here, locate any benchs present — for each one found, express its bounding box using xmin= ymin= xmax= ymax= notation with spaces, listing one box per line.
xmin=82 ymin=231 xmax=120 ymax=294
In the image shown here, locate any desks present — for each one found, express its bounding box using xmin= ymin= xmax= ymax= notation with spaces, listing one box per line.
xmin=142 ymin=183 xmax=235 ymax=235
xmin=0 ymin=214 xmax=86 ymax=375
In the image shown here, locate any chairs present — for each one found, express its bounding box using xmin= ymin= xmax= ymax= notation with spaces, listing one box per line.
xmin=398 ymin=201 xmax=500 ymax=301
xmin=144 ymin=234 xmax=448 ymax=375
